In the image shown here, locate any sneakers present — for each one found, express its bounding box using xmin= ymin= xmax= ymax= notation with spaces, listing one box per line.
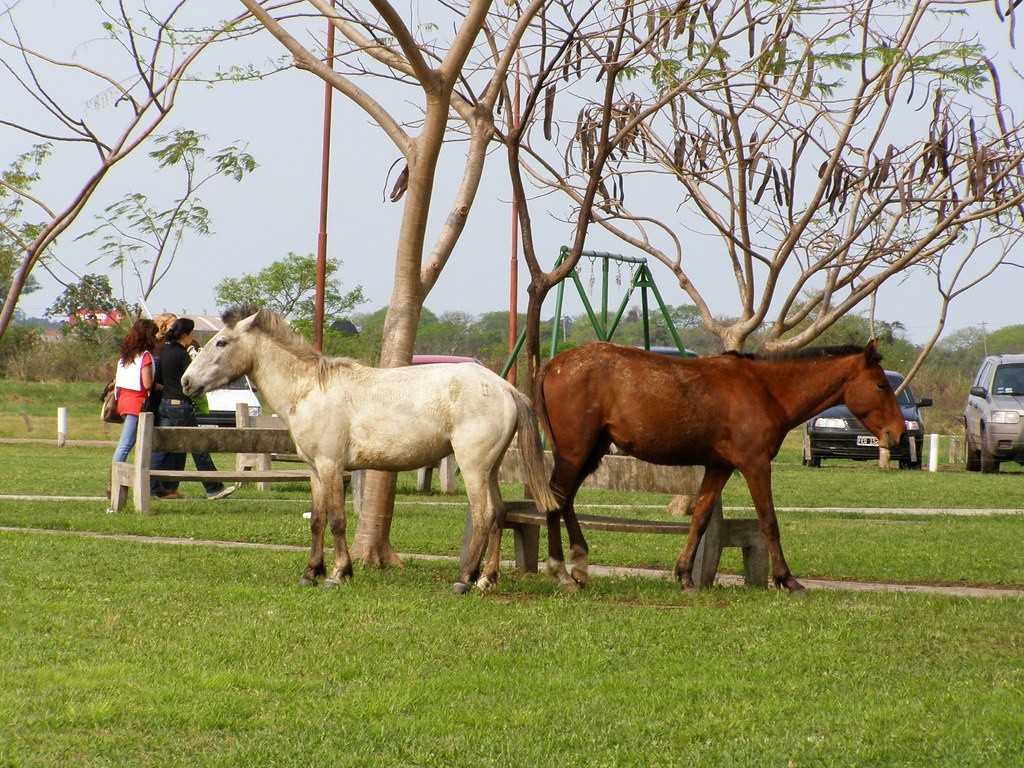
xmin=207 ymin=486 xmax=236 ymax=500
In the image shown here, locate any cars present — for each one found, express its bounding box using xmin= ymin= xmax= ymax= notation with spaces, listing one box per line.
xmin=801 ymin=370 xmax=933 ymax=469
xmin=412 ymin=355 xmax=482 ymax=366
xmin=197 ymin=373 xmax=263 ymax=428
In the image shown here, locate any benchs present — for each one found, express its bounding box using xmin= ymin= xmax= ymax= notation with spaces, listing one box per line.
xmin=235 ymin=403 xmax=456 ymax=493
xmin=110 ymin=412 xmax=353 ymax=514
xmin=460 ymin=500 xmax=768 ymax=589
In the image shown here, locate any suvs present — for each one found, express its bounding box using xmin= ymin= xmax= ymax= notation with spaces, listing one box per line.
xmin=963 ymin=354 xmax=1024 ymax=474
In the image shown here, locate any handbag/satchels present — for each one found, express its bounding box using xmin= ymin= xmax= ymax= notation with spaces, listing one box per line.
xmin=141 ymin=390 xmax=162 ymax=426
xmin=100 ymin=379 xmax=124 ymax=424
xmin=192 ymin=396 xmax=210 ymax=415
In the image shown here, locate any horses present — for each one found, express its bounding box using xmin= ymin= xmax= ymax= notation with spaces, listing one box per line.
xmin=181 ymin=300 xmax=565 ymax=595
xmin=536 ymin=337 xmax=908 ymax=596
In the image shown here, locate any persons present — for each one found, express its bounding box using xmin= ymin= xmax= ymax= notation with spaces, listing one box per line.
xmin=149 ymin=318 xmax=240 ymax=499
xmin=103 ymin=317 xmax=165 ymax=514
xmin=150 ymin=313 xmax=181 ymax=410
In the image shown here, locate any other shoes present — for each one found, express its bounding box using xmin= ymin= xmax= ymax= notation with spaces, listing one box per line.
xmin=162 ymin=494 xmax=177 ymax=499
xmin=171 ymin=490 xmax=185 ymax=498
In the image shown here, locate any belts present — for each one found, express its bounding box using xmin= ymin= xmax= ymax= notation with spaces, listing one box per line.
xmin=164 ymin=396 xmax=188 ymax=399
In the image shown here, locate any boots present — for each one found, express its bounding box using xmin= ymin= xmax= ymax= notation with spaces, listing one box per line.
xmin=106 ymin=469 xmax=112 ymax=499
